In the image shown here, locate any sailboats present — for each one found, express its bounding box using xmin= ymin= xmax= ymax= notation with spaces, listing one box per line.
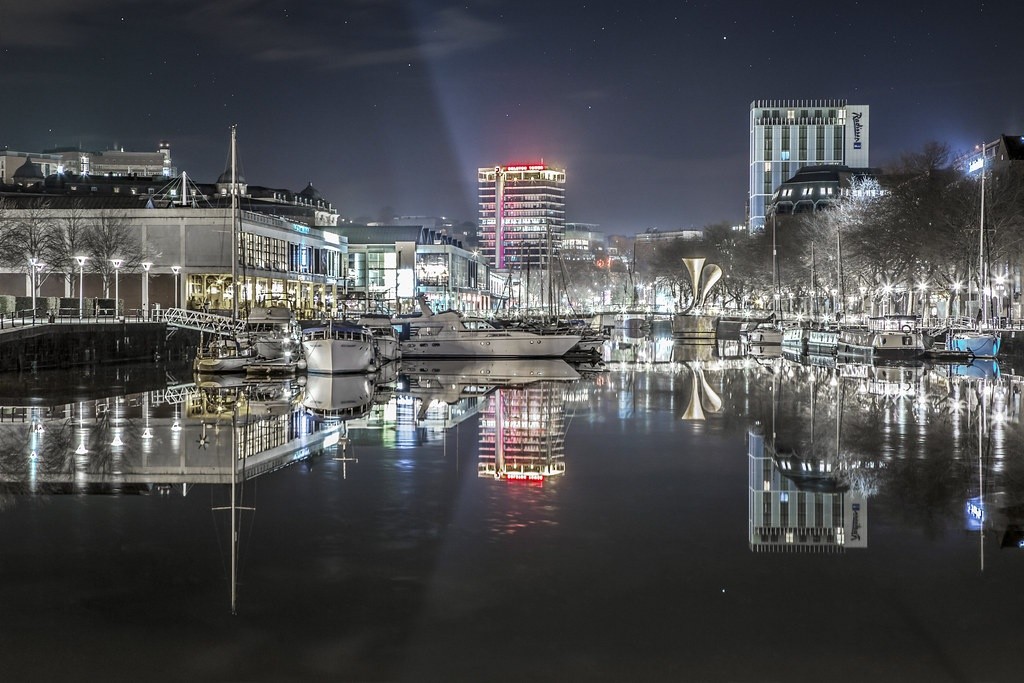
xmin=757 ymin=213 xmax=809 ymax=342
xmin=934 ymin=356 xmax=1002 ymax=572
xmin=807 ymin=240 xmax=842 ymax=348
xmin=806 ymin=342 xmax=837 ymax=444
xmin=837 ymin=226 xmax=872 ymax=348
xmin=945 ymin=141 xmax=1002 ymax=358
xmin=487 ymin=223 xmax=612 ymax=351
xmin=834 ymin=341 xmax=876 ymax=459
xmin=194 ymin=123 xmax=303 ymax=375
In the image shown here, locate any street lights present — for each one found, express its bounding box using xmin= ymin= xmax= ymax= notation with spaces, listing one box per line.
xmin=884 ymin=286 xmax=892 ymax=314
xmin=140 ymin=262 xmax=182 ymax=319
xmin=69 ymin=252 xmax=90 ymax=321
xmin=997 ymin=277 xmax=1004 ymax=328
xmin=953 ymin=283 xmax=960 ymax=321
xmin=76 ymin=388 xmax=183 ymax=454
xmin=919 ymin=284 xmax=927 ymax=311
xmin=108 ymin=258 xmax=125 ymax=319
xmin=30 ymin=256 xmax=48 ymax=320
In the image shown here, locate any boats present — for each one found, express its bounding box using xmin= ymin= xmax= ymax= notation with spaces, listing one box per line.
xmin=356 ymin=288 xmax=590 ymax=359
xmin=374 ymin=359 xmax=582 ymax=421
xmin=356 ymin=325 xmax=402 ymax=360
xmin=301 ymin=316 xmax=375 ymax=374
xmin=747 ymin=322 xmax=783 ymax=344
xmin=747 ymin=341 xmax=783 ymax=367
